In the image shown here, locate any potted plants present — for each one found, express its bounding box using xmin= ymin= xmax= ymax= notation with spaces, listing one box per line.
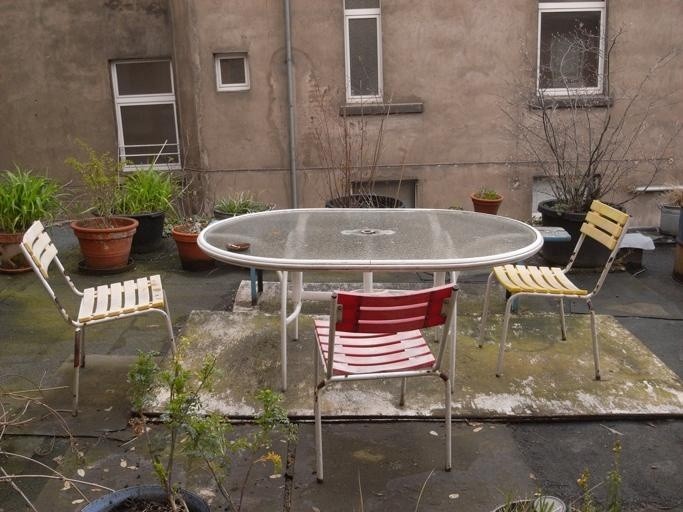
xmin=76 ymin=352 xmax=292 ymax=512
xmin=213 ymin=194 xmax=278 ymax=223
xmin=166 ymin=137 xmax=218 ymax=272
xmin=470 ymin=185 xmax=504 ymax=217
xmin=307 ymin=80 xmax=408 ymax=212
xmin=488 ymin=15 xmax=682 ymax=270
xmin=59 ymin=145 xmax=141 ymax=279
xmin=0 ymin=157 xmax=55 ymax=275
xmin=655 ymin=168 xmax=682 ymax=236
xmin=490 ymin=495 xmax=569 ymax=512
xmin=115 ymin=161 xmax=178 ymax=255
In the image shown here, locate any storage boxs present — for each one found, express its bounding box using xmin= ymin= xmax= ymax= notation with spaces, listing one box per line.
xmin=618 ymin=241 xmax=644 ymax=268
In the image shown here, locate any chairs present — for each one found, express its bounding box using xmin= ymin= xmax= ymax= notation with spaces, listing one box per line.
xmin=17 ymin=217 xmax=185 ymax=420
xmin=470 ymin=196 xmax=633 ymax=385
xmin=309 ymin=277 xmax=462 ymax=485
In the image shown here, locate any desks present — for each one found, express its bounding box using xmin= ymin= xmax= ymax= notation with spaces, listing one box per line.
xmin=193 ymin=206 xmax=550 ymax=395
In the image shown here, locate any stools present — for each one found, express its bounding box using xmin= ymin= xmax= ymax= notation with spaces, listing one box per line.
xmin=532 ymin=224 xmax=573 ymax=244
